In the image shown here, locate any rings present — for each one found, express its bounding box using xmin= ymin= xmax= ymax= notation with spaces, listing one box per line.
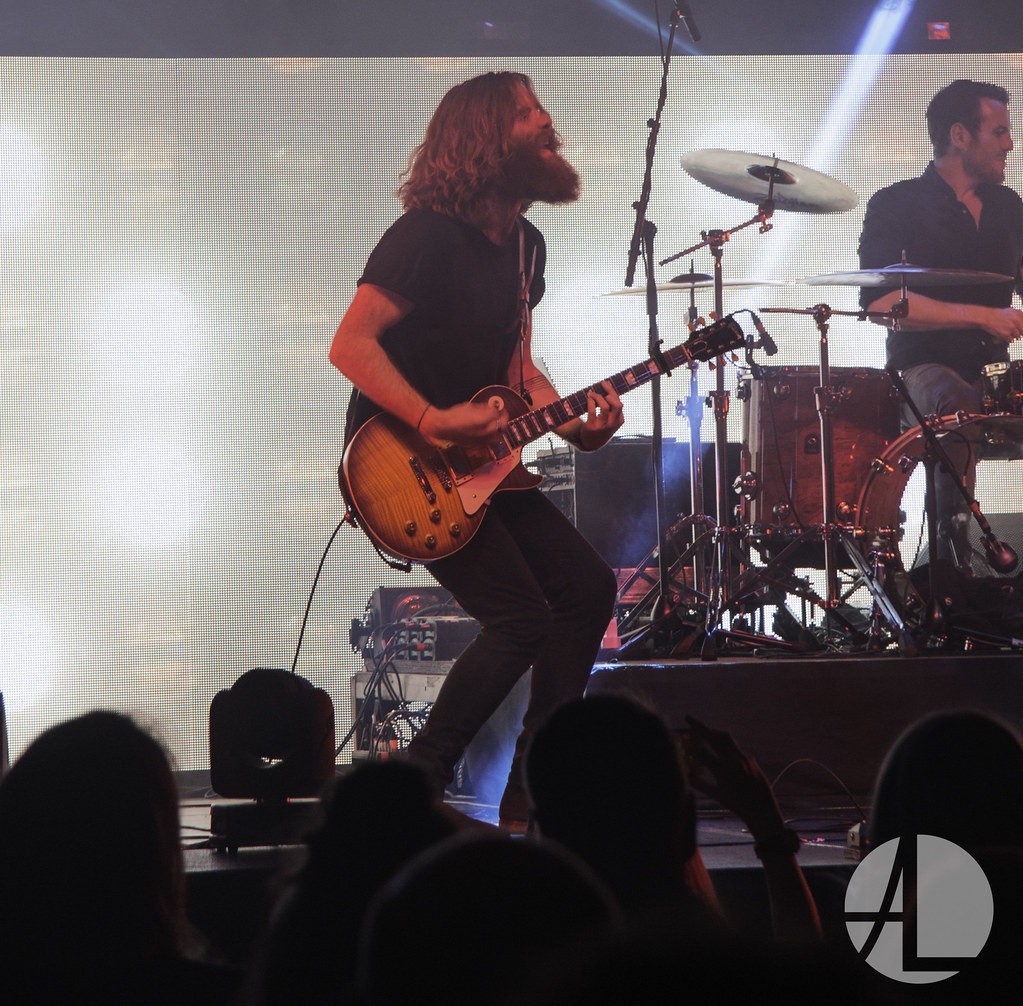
xmin=497 ymin=419 xmax=502 ymax=432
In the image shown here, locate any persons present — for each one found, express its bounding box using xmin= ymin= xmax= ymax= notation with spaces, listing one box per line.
xmin=329 ymin=71 xmax=624 ymax=838
xmin=0 ymin=694 xmax=1023 ymax=1006
xmin=856 ymin=79 xmax=1023 ymax=580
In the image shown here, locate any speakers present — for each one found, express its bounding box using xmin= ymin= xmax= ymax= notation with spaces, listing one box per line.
xmin=362 ymin=586 xmax=461 ymax=659
xmin=569 ymin=440 xmax=749 ymax=571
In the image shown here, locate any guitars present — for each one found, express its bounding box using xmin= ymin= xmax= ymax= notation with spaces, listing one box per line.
xmin=337 ymin=310 xmax=747 ymax=565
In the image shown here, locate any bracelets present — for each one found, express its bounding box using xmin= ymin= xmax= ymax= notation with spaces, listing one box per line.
xmin=416 ymin=403 xmax=430 ymax=431
xmin=576 ymin=430 xmax=587 ymax=450
xmin=753 ymin=829 xmax=799 ymax=859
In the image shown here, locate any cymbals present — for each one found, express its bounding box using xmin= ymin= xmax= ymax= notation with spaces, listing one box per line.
xmin=794 ymin=262 xmax=1016 ymax=288
xmin=598 ymin=274 xmax=786 ymax=298
xmin=680 ymin=148 xmax=860 ymax=214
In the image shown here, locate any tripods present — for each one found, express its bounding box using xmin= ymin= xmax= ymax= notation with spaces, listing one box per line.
xmin=614 ymin=9 xmax=1023 ymax=660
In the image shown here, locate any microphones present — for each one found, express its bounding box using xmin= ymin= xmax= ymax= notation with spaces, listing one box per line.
xmin=750 ymin=312 xmax=778 ymax=356
xmin=985 ymin=530 xmax=1017 ymax=572
xmin=673 ymin=0 xmax=701 ymax=42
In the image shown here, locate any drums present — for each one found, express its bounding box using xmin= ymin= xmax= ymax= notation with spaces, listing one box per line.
xmin=853 ymin=409 xmax=1023 ymax=651
xmin=979 ymin=360 xmax=1023 ymax=451
xmin=736 ymin=364 xmax=904 ymax=540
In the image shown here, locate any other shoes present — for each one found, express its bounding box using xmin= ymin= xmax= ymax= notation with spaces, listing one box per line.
xmin=494 ymin=813 xmax=533 ymax=840
